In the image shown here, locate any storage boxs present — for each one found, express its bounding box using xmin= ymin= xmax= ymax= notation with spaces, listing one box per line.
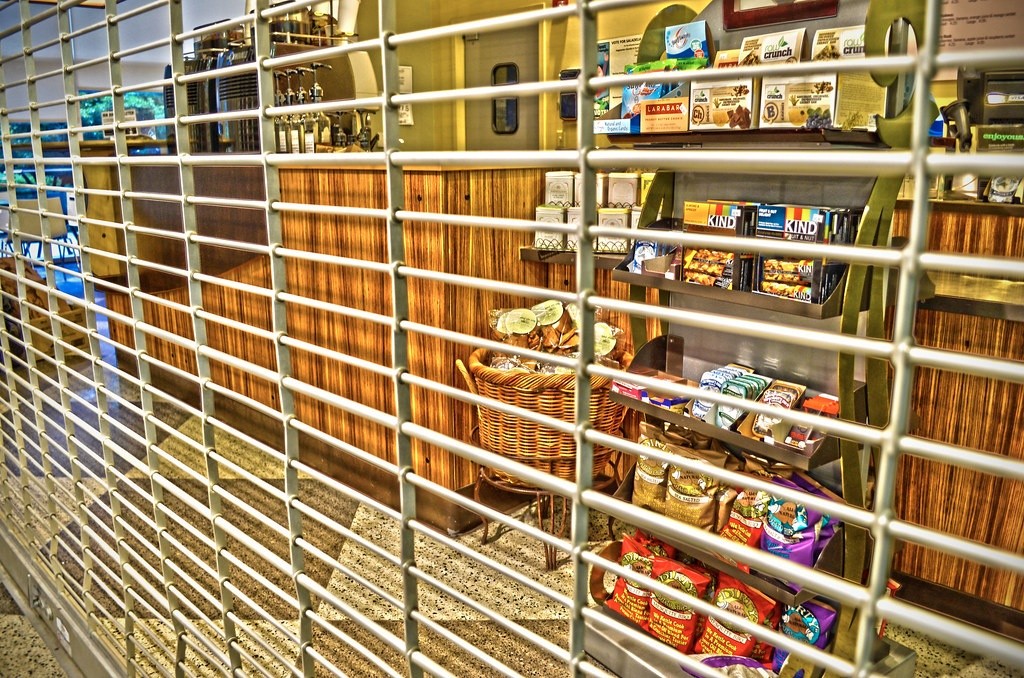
xmin=0 ymin=257 xmax=90 ymax=362
xmin=680 ymin=196 xmax=864 ymax=308
xmin=593 ymin=19 xmax=885 ymax=132
xmin=533 ymin=171 xmax=665 ymax=254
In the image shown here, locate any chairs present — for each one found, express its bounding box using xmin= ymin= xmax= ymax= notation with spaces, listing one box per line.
xmin=0 ymin=191 xmax=82 ymax=282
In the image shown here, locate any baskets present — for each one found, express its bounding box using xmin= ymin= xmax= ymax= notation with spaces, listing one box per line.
xmin=467 ymin=348 xmax=631 ymax=488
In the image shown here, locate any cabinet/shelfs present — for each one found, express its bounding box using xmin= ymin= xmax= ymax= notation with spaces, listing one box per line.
xmin=582 ymin=0 xmax=939 ymax=678
